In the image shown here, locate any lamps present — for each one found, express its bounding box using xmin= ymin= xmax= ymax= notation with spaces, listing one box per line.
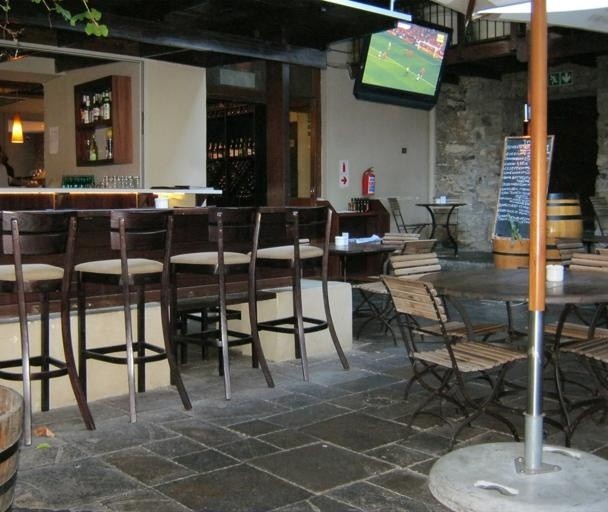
xmin=11 ymin=82 xmax=24 ymax=143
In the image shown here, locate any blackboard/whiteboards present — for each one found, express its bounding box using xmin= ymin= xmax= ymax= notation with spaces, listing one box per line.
xmin=489 ymin=135 xmax=555 ymax=241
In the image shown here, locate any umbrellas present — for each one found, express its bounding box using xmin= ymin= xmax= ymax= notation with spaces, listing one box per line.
xmin=403 ymin=1 xmax=608 ymax=415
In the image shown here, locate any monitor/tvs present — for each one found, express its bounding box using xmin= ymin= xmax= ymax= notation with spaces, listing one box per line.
xmin=352 ymin=18 xmax=453 ymax=112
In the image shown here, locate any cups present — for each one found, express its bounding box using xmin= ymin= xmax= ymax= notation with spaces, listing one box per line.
xmin=335 ymin=235 xmax=344 ymax=246
xmin=433 ymin=196 xmax=441 ymax=204
xmin=544 ymin=263 xmax=564 ymax=283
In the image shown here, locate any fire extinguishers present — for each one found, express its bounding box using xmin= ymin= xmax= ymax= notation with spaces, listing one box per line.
xmin=361 ymin=167 xmax=375 ymax=196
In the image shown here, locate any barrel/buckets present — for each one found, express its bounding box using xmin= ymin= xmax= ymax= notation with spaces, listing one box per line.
xmin=1 ymin=385 xmax=24 ymax=512
xmin=546 ymin=192 xmax=584 ymax=265
xmin=493 ymin=237 xmax=529 ymax=269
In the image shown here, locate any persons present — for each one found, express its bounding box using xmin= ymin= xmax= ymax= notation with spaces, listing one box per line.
xmin=378 ymin=19 xmax=448 ymax=82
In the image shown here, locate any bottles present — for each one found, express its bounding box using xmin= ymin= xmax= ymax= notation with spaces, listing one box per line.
xmin=206 ymin=134 xmax=256 ymax=159
xmin=78 ymin=87 xmax=112 ymax=124
xmin=85 ymin=135 xmax=96 ymax=160
xmin=94 ymin=175 xmax=139 ymax=188
xmin=104 ymin=129 xmax=113 ymax=159
xmin=60 ymin=174 xmax=95 ymax=188
xmin=348 ymin=197 xmax=371 ymax=213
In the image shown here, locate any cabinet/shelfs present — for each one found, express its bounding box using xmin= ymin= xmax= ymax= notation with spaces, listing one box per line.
xmin=206 ymin=102 xmax=265 ymax=207
xmin=73 ymin=74 xmax=133 ymax=168
